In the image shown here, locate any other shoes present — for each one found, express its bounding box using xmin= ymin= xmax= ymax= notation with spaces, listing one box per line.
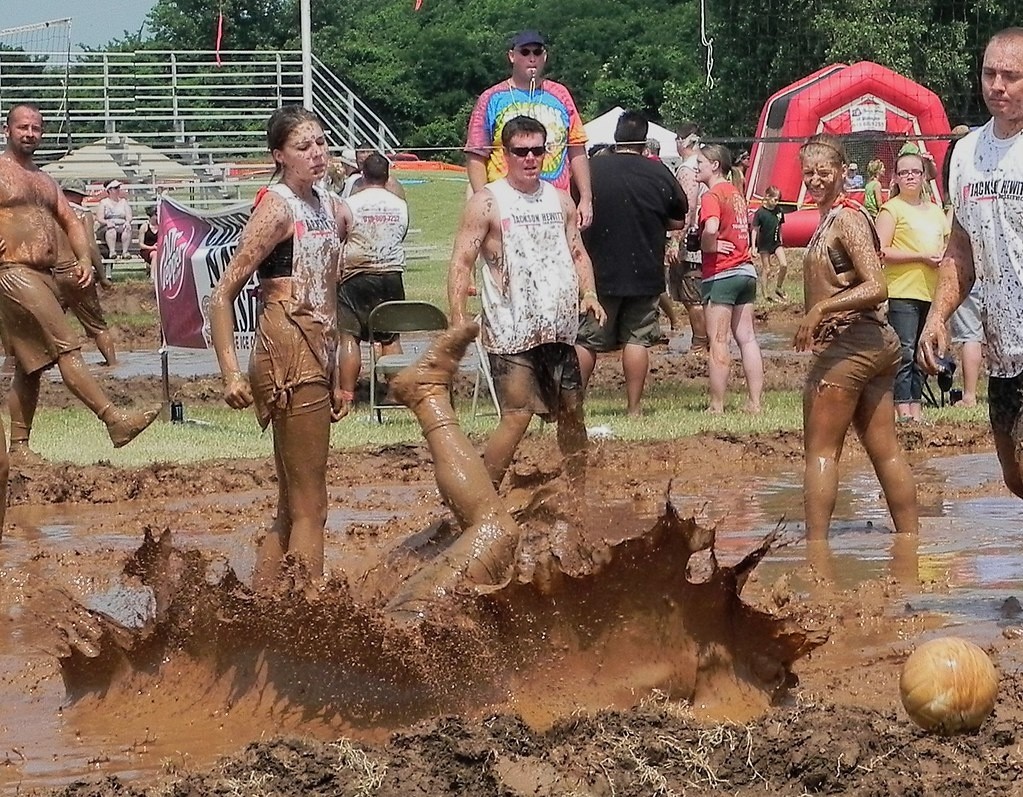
xmin=109 ymin=404 xmax=162 ymax=448
xmin=109 ymin=252 xmax=117 ymax=259
xmin=121 ymin=253 xmax=132 ymax=259
xmin=392 ymin=322 xmax=480 ymax=410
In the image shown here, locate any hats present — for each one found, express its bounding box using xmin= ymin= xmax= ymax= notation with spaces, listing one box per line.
xmin=511 ymin=33 xmax=546 ymax=49
xmin=60 ymin=177 xmax=91 ymax=197
xmin=849 ymin=163 xmax=858 ymax=171
xmin=105 ymin=180 xmax=122 ymax=191
xmin=338 ymin=149 xmax=357 ymax=169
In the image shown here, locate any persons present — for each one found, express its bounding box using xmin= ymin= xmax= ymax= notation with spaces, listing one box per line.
xmin=463 ymin=29 xmax=593 ymax=233
xmin=95 ymin=179 xmax=132 ymax=259
xmin=137 ymin=205 xmax=158 ymax=279
xmin=572 ymin=112 xmax=764 ymax=415
xmin=206 ymin=106 xmax=343 ymax=590
xmin=750 ymin=186 xmax=789 ymax=299
xmin=876 ymin=123 xmax=987 ymax=418
xmin=920 ymin=25 xmax=1023 ymax=498
xmin=792 ymin=135 xmax=918 ymax=538
xmin=0 ymin=106 xmax=161 ymax=465
xmin=361 ymin=321 xmax=519 ymax=629
xmin=330 ymin=142 xmax=410 ymax=408
xmin=51 ymin=178 xmax=118 ymax=366
xmin=447 ymin=114 xmax=608 ymax=493
xmin=863 ymin=158 xmax=885 ymax=218
xmin=846 ymin=162 xmax=863 ymax=189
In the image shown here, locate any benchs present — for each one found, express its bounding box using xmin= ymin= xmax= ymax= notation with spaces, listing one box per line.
xmin=60 ymin=183 xmax=271 ymax=279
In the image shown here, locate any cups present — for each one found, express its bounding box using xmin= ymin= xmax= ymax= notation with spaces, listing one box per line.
xmin=950 ymin=389 xmax=962 ymax=406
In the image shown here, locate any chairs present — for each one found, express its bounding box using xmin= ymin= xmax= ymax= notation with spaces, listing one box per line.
xmin=368 ymin=301 xmax=454 ymax=425
xmin=473 ymin=314 xmax=501 ymax=420
xmin=918 ymin=348 xmax=957 ymax=408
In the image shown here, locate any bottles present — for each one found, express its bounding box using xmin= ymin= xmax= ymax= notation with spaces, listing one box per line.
xmin=171 ymin=401 xmax=183 ymax=425
xmin=686 ymin=227 xmax=700 ymax=252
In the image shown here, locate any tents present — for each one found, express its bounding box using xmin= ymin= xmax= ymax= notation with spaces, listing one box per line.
xmin=582 ymin=106 xmax=705 ymax=159
xmin=42 ymin=131 xmax=195 ymax=208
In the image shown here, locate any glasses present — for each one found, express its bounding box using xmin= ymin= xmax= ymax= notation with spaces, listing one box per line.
xmin=112 ymin=187 xmax=120 ymax=189
xmin=514 ymin=47 xmax=544 ymax=56
xmin=896 ymin=169 xmax=924 ymax=178
xmin=742 ymin=157 xmax=750 ymax=160
xmin=508 ymin=146 xmax=546 ymax=157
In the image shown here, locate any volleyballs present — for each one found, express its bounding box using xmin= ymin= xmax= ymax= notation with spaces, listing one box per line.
xmin=899 ymin=636 xmax=998 ymax=737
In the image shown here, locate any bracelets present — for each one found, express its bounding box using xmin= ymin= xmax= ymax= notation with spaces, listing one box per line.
xmin=583 ymin=291 xmax=598 ymax=299
